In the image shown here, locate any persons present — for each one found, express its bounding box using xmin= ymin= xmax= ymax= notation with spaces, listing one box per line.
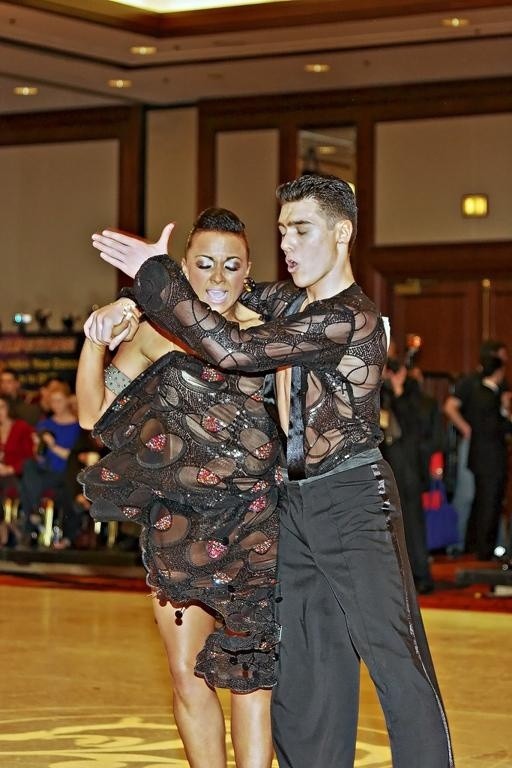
xmin=381 ymin=336 xmax=512 ymax=592
xmin=76 ymin=206 xmax=284 ymax=767
xmin=1 ymin=368 xmax=142 ymax=552
xmin=90 ymin=171 xmax=455 ymax=766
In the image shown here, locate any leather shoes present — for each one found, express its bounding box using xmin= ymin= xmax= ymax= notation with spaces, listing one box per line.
xmin=414 ymin=571 xmax=433 ymax=593
